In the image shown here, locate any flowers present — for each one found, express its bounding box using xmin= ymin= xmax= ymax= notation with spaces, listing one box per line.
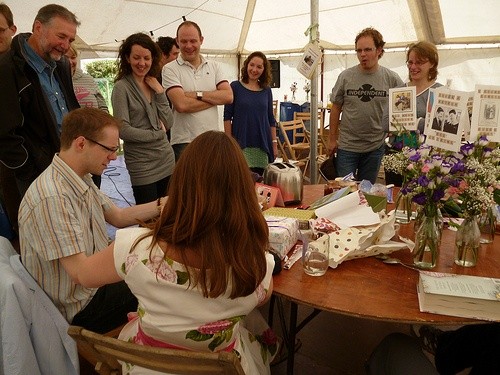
xmin=378 ymin=114 xmax=500 ymax=267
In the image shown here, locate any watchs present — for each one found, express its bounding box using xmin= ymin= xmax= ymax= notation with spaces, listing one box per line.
xmin=197 ymin=91 xmax=203 ymax=101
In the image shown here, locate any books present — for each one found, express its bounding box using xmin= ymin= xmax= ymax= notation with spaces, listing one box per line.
xmin=261 ymin=207 xmax=316 ymax=230
xmin=364 ymin=194 xmax=387 ymax=212
xmin=417 ymin=271 xmax=500 ymax=322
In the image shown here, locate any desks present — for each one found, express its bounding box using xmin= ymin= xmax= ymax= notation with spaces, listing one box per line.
xmin=140 ymin=180 xmax=500 ymax=375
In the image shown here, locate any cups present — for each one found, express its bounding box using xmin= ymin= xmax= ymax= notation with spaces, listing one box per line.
xmin=303 ymin=231 xmax=330 ymax=276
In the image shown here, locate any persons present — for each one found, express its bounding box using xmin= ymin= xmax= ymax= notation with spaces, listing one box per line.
xmin=383 ymin=41 xmax=445 ymax=187
xmin=20 ymin=110 xmax=169 ymax=334
xmin=64 ymin=43 xmax=109 ymax=190
xmin=78 ymin=130 xmax=279 ymax=375
xmin=305 ymin=55 xmax=311 ymax=63
xmin=224 ymin=51 xmax=278 ymax=177
xmin=395 ymin=93 xmax=408 ymax=107
xmin=444 ymin=109 xmax=459 ymax=135
xmin=326 ymin=26 xmax=405 ymax=185
xmin=112 ymin=22 xmax=233 ymax=227
xmin=0 ymin=3 xmax=80 ymax=249
xmin=432 ymin=106 xmax=444 ymax=131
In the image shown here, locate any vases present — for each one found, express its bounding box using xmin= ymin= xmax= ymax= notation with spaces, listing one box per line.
xmin=476 ymin=202 xmax=495 ymax=244
xmin=394 ymin=187 xmax=410 ymax=225
xmin=414 ymin=200 xmax=444 ymax=252
xmin=453 ymin=211 xmax=481 ymax=269
xmin=413 ymin=215 xmax=439 ymax=269
xmin=410 ymin=200 xmax=425 ymax=221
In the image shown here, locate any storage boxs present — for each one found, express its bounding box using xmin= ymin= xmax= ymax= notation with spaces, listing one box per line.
xmin=364 ymin=194 xmax=386 ymax=218
xmin=261 ymin=206 xmax=316 ymax=261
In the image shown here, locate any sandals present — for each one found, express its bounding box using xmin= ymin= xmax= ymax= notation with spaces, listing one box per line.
xmin=269 ymin=337 xmax=302 ymax=365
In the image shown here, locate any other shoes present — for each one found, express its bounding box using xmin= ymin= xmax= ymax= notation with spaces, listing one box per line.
xmin=410 ymin=321 xmax=442 ymax=354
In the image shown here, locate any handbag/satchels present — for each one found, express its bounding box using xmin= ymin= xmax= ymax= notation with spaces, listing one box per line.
xmin=319 ymin=154 xmax=336 ymax=181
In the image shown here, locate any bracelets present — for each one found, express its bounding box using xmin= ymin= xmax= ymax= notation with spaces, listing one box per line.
xmin=157 ymin=198 xmax=161 ymax=217
xmin=273 ymin=140 xmax=277 ymax=143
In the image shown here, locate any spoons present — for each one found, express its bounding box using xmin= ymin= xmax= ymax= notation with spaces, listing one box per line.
xmin=376 ymin=256 xmax=428 ymax=271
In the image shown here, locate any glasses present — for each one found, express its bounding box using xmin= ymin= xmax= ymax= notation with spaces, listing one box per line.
xmin=74 ymin=135 xmax=119 ymax=154
xmin=406 ymin=59 xmax=429 ymax=66
xmin=355 ymin=47 xmax=376 ymax=53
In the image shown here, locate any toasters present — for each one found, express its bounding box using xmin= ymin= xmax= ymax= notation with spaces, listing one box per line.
xmin=262 ymin=162 xmax=303 ymax=207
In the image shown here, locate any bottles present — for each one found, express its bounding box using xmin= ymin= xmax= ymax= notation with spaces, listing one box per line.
xmin=324 ymin=181 xmax=341 ymax=196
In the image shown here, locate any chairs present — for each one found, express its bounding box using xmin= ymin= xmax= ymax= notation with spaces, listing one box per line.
xmin=272 ymin=98 xmax=345 ymax=185
xmin=0 ymin=236 xmax=128 ymax=375
xmin=67 ymin=323 xmax=246 ymax=375
